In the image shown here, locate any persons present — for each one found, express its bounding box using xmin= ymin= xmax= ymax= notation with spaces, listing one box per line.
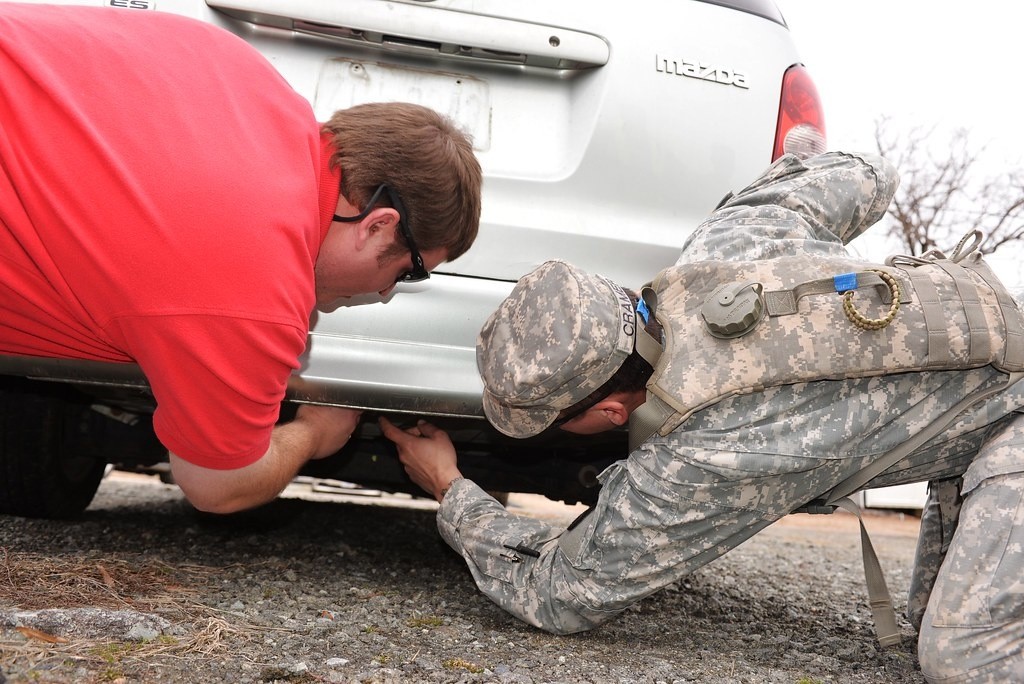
xmin=375 ymin=149 xmax=1024 ymax=684
xmin=0 ymin=1 xmax=484 ymax=513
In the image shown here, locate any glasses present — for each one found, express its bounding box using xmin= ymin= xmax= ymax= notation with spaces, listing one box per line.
xmin=380 ymin=181 xmax=429 ymax=282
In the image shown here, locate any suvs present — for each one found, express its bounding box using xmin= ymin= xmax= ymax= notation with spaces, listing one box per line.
xmin=0 ymin=2 xmax=824 ymax=524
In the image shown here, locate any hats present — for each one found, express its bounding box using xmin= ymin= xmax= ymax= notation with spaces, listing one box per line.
xmin=474 ymin=260 xmax=635 ymax=439
